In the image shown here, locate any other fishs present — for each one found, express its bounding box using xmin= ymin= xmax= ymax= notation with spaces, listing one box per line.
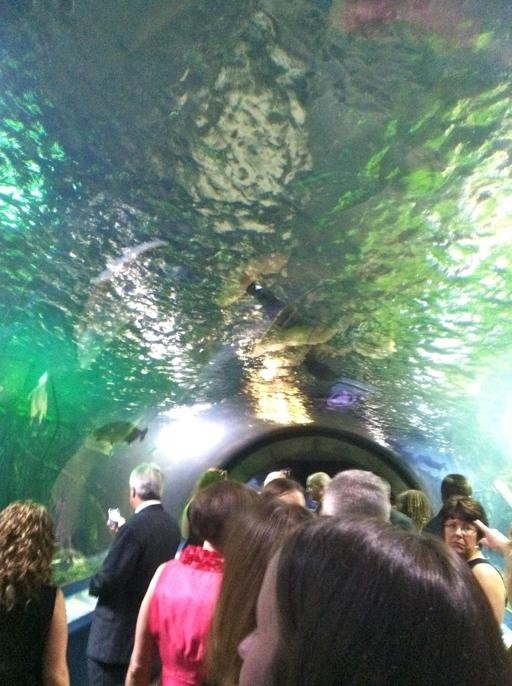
xmin=384 ymin=437 xmax=448 ymax=482
xmin=93 ymin=420 xmax=149 ymax=445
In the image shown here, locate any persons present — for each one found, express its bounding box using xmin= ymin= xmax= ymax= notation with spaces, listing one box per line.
xmin=261 ymin=468 xmax=391 ymax=524
xmin=84 ymin=464 xmax=182 ymax=683
xmin=0 ymin=499 xmax=71 ymax=685
xmin=125 ymin=469 xmax=315 ymax=683
xmin=390 ymin=473 xmax=510 ymax=627
xmin=239 ymin=514 xmax=510 ymax=684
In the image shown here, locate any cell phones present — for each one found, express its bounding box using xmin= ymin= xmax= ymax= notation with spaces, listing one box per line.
xmin=108 ymin=508 xmax=120 ymax=529
